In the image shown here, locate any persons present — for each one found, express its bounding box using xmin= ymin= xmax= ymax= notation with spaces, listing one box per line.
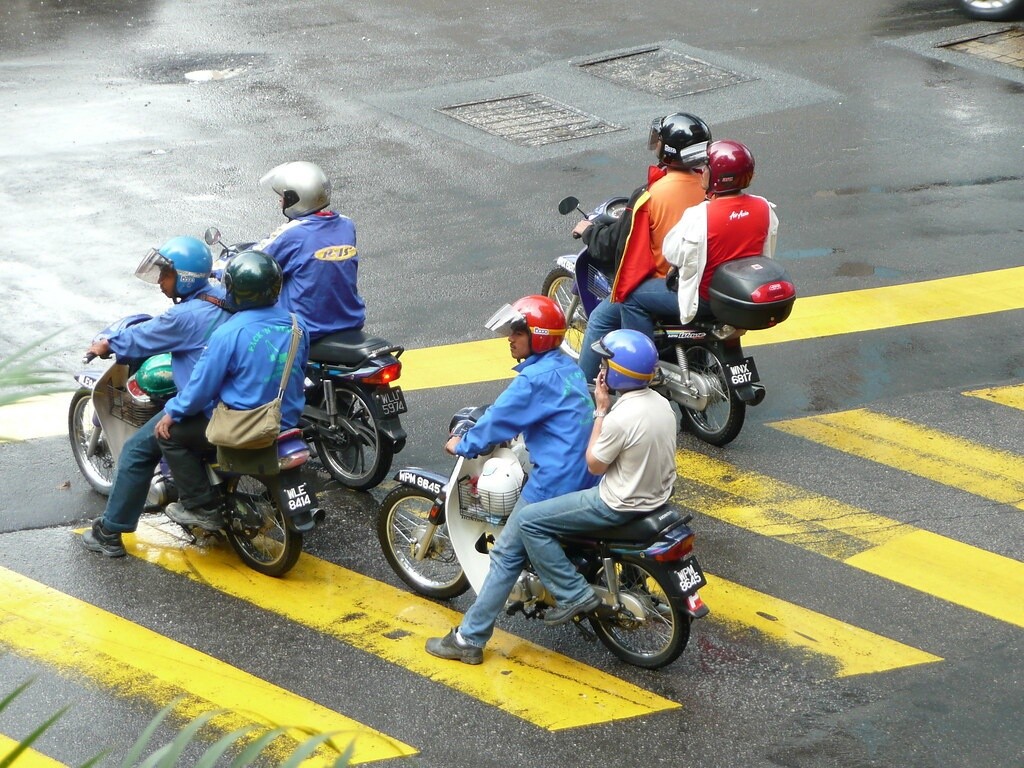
xmin=154 ymin=250 xmax=310 ymax=531
xmin=517 ymin=328 xmax=677 ymax=627
xmin=214 ymin=161 xmax=367 ymax=342
xmin=81 ymin=236 xmax=233 ymax=560
xmin=620 ymin=139 xmax=779 ymax=340
xmin=425 ymin=295 xmax=604 ymax=665
xmin=570 ymin=112 xmax=712 ymax=386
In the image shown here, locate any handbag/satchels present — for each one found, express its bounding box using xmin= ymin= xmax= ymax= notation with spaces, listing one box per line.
xmin=204 ymin=397 xmax=282 ymax=449
xmin=666 ymin=267 xmax=678 ymax=292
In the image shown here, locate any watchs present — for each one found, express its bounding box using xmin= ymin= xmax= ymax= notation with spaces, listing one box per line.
xmin=593 ymin=410 xmax=605 ymax=418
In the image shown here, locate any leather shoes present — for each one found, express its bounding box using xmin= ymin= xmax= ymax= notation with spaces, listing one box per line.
xmin=424 ymin=625 xmax=483 ymax=665
xmin=543 ymin=592 xmax=603 ymax=626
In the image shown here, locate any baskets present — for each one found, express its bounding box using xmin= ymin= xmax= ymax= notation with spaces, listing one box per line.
xmin=106 ymin=376 xmax=164 ymax=428
xmin=457 ymin=475 xmax=528 ymax=526
xmin=588 ymin=264 xmax=614 ymax=300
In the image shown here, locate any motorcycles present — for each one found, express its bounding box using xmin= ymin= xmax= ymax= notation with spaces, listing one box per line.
xmin=541 ymin=196 xmax=796 ymax=446
xmin=68 ymin=313 xmax=327 ymax=579
xmin=205 ymin=226 xmax=408 ymax=493
xmin=377 ymin=404 xmax=709 ymax=670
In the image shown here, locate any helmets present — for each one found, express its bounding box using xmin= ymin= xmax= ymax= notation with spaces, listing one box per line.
xmin=135 ymin=354 xmax=177 ymax=398
xmin=700 ymin=140 xmax=755 ymax=194
xmin=503 ymin=295 xmax=566 ymax=354
xmin=148 ymin=237 xmax=213 ymax=296
xmin=271 ymin=161 xmax=331 ymax=219
xmin=225 ymin=250 xmax=283 ymax=311
xmin=590 ymin=328 xmax=659 ymax=391
xmin=652 ymin=112 xmax=713 ymax=168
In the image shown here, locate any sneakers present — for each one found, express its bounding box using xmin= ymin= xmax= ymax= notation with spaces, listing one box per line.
xmin=81 ymin=518 xmax=126 ymax=557
xmin=165 ymin=502 xmax=225 ymax=531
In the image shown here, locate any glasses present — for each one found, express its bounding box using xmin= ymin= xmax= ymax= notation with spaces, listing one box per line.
xmin=599 ymin=364 xmax=607 ymax=369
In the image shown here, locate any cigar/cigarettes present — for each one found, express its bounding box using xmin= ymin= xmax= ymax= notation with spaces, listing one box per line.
xmin=593 ymin=378 xmax=603 ymax=382
